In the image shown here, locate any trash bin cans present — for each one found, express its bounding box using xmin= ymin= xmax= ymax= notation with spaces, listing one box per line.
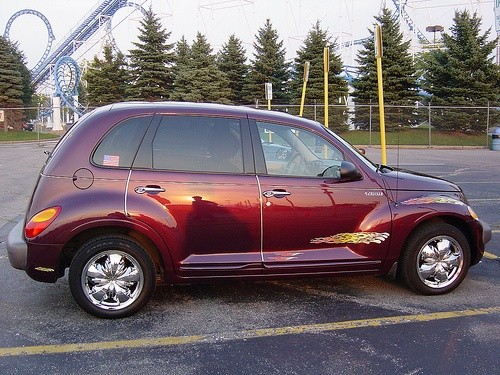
xmin=490 ymin=127 xmax=500 ymax=152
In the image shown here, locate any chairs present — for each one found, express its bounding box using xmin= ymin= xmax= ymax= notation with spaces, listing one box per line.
xmin=200 ymin=128 xmax=242 ymax=176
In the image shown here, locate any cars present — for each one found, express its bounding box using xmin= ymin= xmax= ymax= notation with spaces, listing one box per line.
xmin=9 ymin=103 xmax=491 ymax=318
